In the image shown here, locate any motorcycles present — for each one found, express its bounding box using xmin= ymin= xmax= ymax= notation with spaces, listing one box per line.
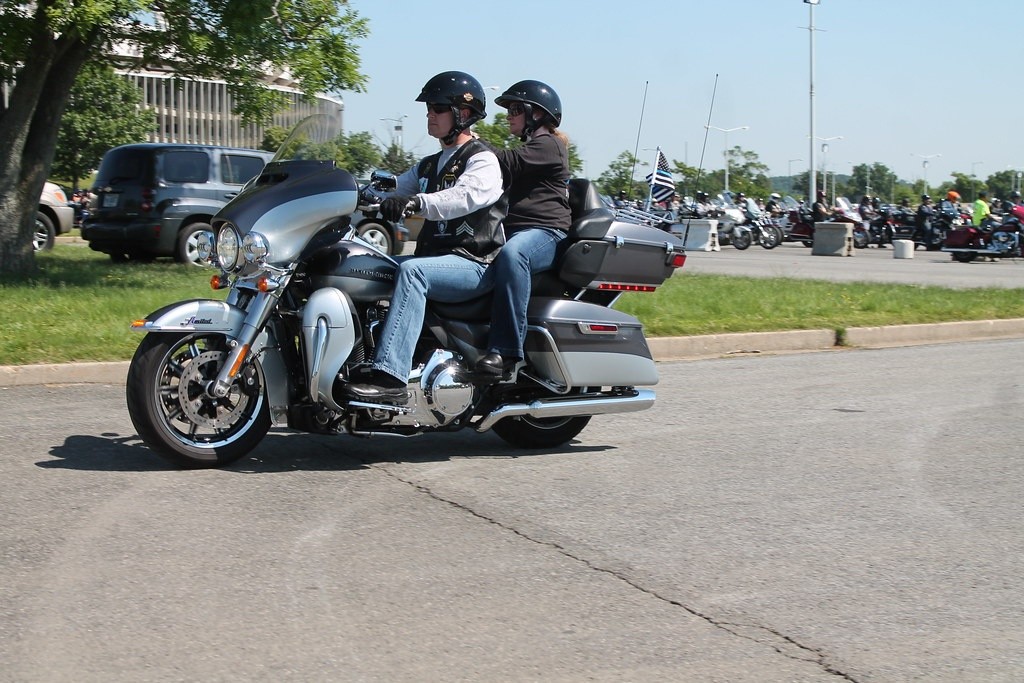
xmin=124 ymin=114 xmax=690 ymax=470
xmin=596 ymin=193 xmax=1023 ymax=262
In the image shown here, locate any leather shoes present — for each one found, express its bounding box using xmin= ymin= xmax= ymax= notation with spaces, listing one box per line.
xmin=478 ymin=352 xmax=515 ymax=374
xmin=343 ymin=373 xmax=408 ymax=407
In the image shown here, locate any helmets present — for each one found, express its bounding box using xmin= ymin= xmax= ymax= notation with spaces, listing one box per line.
xmin=1009 ymin=190 xmax=1022 ymax=198
xmin=947 ymin=191 xmax=962 ymax=202
xmin=493 ymin=79 xmax=562 ymax=127
xmin=416 ymin=70 xmax=487 ymax=117
xmin=618 ymin=189 xmax=934 ymax=206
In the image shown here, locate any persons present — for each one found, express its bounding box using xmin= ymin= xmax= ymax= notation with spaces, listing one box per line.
xmin=340 ymin=71 xmax=513 ymax=404
xmin=470 ymin=80 xmax=575 ymax=375
xmin=69 ymin=189 xmax=92 ymax=227
xmin=615 ymin=189 xmax=1024 ymax=262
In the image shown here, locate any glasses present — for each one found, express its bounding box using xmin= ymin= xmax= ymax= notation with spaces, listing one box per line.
xmin=507 ymin=104 xmax=526 ymax=117
xmin=425 ymin=102 xmax=460 ymax=114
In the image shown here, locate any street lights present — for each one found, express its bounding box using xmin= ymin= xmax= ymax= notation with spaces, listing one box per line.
xmin=971 ymin=161 xmax=983 ymax=202
xmin=804 ymin=0 xmax=821 ymax=208
xmin=826 ymin=162 xmax=851 ymax=205
xmin=866 ymin=164 xmax=885 ymax=194
xmin=788 ymin=159 xmax=802 ymax=193
xmin=805 ymin=136 xmax=845 ymax=200
xmin=706 ymin=125 xmax=749 ymax=191
xmin=910 ymin=153 xmax=942 ymax=194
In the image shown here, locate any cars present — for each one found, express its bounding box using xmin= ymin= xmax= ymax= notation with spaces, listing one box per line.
xmin=348 ymin=204 xmax=427 ymax=256
xmin=32 ymin=183 xmax=74 ymax=252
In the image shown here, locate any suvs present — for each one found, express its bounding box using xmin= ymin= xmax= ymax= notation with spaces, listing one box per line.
xmin=82 ymin=141 xmax=283 ymax=271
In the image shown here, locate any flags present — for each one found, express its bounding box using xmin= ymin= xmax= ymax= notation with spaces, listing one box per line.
xmin=646 ymin=151 xmax=675 ymax=204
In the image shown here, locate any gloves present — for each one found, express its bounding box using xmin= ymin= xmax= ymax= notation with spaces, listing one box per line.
xmin=380 ymin=194 xmax=421 ymax=224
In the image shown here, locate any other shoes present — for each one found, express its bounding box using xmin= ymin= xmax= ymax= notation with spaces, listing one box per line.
xmin=984 ymin=256 xmax=999 ymax=262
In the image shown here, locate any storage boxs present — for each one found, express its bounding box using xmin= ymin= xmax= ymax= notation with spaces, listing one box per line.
xmin=557 ymin=209 xmax=686 ymax=292
xmin=524 ymin=297 xmax=658 ymax=386
